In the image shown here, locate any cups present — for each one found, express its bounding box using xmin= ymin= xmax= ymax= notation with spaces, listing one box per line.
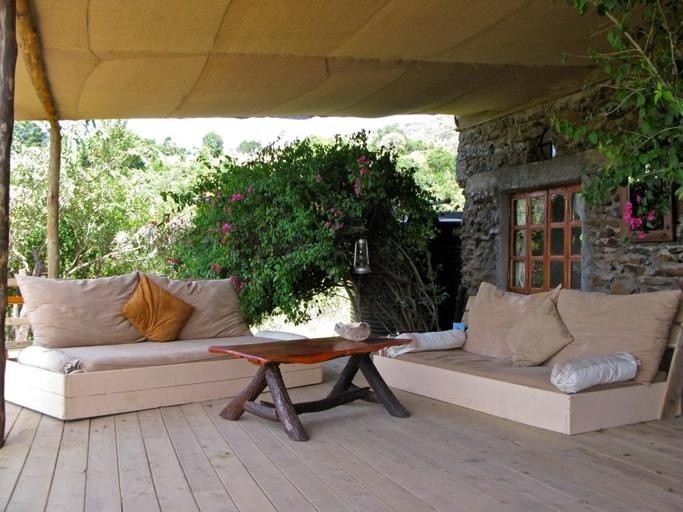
xmin=386 ymin=333 xmax=399 ymax=358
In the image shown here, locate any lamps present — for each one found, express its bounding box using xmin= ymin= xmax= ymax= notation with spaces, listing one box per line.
xmin=352 ymin=226 xmax=371 ymax=322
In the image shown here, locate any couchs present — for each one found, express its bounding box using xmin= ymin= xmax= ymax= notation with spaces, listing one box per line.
xmin=369 ymin=296 xmax=683 ymax=436
xmin=3 ymin=279 xmax=323 ymax=420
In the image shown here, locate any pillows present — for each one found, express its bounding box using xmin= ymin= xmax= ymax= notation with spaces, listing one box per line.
xmin=13 ymin=272 xmax=309 ymax=372
xmin=386 ymin=281 xmax=683 ymax=394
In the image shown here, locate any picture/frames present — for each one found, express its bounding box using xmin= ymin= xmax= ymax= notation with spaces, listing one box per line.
xmin=619 ymin=183 xmax=674 ymax=243
